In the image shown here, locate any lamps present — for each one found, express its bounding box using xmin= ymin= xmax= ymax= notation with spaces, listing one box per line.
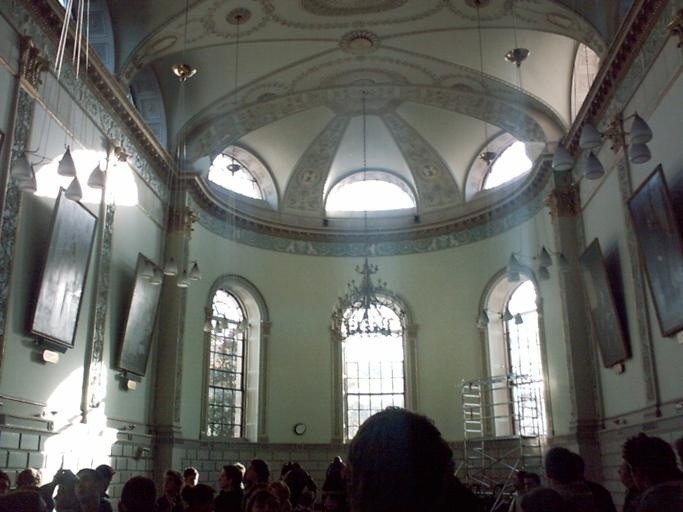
xmin=328 ymin=91 xmax=407 ymax=342
xmin=11 ymin=0 xmax=105 ymax=201
xmin=504 ymin=3 xmax=573 ymax=283
xmin=140 ymin=0 xmax=202 ymax=289
xmin=202 ymin=15 xmax=249 ymax=334
xmin=475 ymin=2 xmax=523 ymax=328
xmin=552 ymin=0 xmax=653 ymax=179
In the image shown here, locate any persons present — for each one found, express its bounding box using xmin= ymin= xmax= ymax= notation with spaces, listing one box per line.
xmin=487 ymin=431 xmax=683 ymax=512
xmin=119 ymin=456 xmax=349 ymax=511
xmin=1 ymin=464 xmax=116 ymax=511
xmin=346 ymin=406 xmax=484 ymax=511
